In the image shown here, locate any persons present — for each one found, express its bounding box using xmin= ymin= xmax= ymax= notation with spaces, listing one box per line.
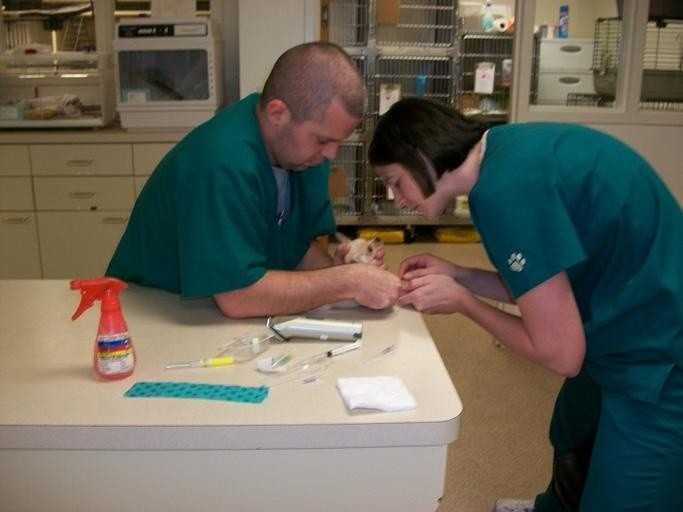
xmin=104 ymin=42 xmax=402 ymax=318
xmin=368 ymin=96 xmax=683 ymax=511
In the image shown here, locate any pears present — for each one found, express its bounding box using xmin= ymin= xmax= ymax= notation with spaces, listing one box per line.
xmin=66 ymin=277 xmax=138 ymax=380
xmin=482 ymin=2 xmax=493 ymax=33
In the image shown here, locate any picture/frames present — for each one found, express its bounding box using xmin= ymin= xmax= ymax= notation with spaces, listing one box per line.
xmin=0 ymin=278 xmax=465 ymax=512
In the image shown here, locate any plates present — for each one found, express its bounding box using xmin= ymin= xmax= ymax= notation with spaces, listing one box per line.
xmin=322 ymin=1 xmax=523 ymax=228
xmin=0 ymin=129 xmax=181 ymax=280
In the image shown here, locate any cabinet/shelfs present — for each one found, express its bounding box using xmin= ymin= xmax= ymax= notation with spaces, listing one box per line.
xmin=559 ymin=5 xmax=569 ymax=38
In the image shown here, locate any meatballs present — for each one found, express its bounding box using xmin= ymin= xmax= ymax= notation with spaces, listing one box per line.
xmin=165 ymin=356 xmax=234 ymax=370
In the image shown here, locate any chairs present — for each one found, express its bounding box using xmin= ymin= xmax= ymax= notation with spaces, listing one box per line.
xmin=496 ymin=498 xmax=535 ymax=512
